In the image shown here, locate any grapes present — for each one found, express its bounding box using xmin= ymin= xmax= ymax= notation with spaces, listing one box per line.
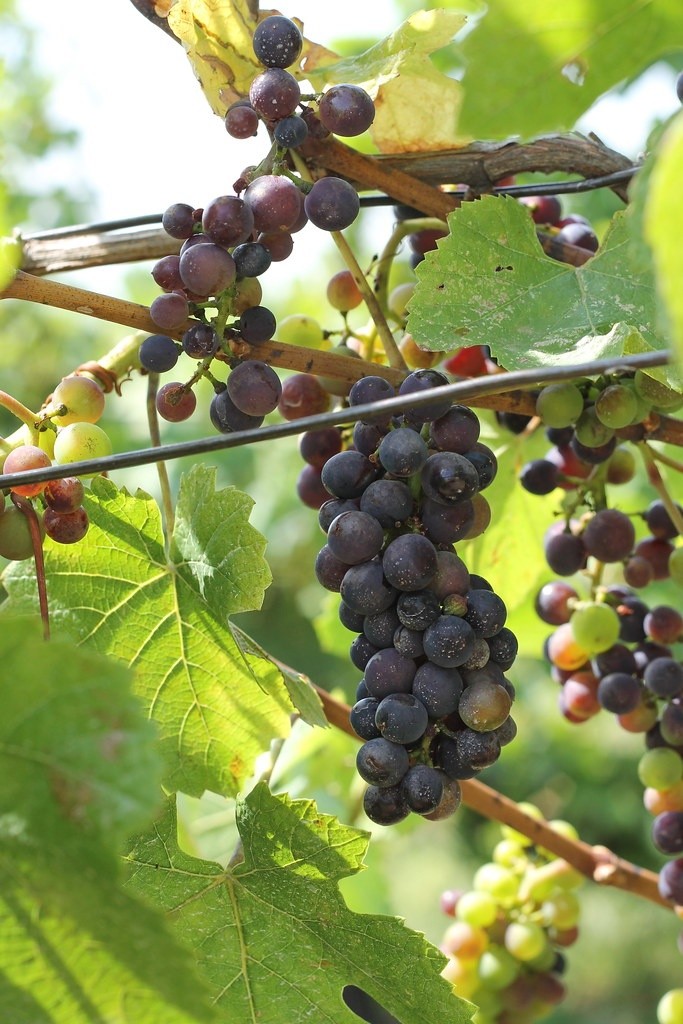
xmin=0 ymin=14 xmax=683 ymax=1024
